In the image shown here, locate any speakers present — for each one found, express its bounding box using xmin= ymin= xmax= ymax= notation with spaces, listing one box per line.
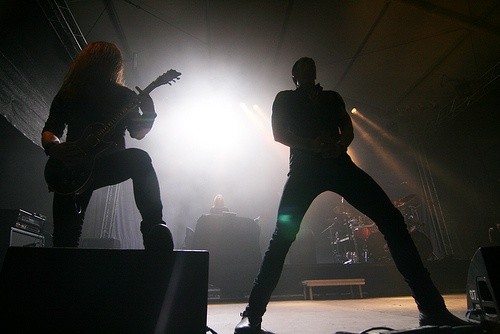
xmin=0 ymin=244 xmax=209 ymax=334
xmin=0 ymin=227 xmax=45 ymax=247
xmin=466 ymin=246 xmax=500 ymax=334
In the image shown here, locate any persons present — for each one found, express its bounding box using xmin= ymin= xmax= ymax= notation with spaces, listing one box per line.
xmin=334 ymin=205 xmax=346 ymax=232
xmin=342 ymin=250 xmax=358 ymax=264
xmin=41 ymin=42 xmax=175 ymax=249
xmin=209 ymin=194 xmax=230 ymax=215
xmin=234 ymin=58 xmax=476 ymax=334
xmin=383 ymin=242 xmax=391 ymax=256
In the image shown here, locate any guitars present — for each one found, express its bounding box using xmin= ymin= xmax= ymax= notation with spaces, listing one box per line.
xmin=44 ymin=69 xmax=181 ymax=196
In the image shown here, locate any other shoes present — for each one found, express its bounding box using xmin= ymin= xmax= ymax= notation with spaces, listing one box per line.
xmin=419 ymin=305 xmax=466 ymax=326
xmin=140 ymin=217 xmax=175 ymax=250
xmin=234 ymin=310 xmax=262 ymax=334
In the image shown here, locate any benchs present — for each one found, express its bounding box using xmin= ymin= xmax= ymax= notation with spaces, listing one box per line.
xmin=301 ymin=278 xmax=366 ymax=300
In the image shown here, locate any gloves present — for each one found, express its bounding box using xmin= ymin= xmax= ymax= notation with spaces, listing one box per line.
xmin=132 ymin=86 xmax=157 ymax=118
